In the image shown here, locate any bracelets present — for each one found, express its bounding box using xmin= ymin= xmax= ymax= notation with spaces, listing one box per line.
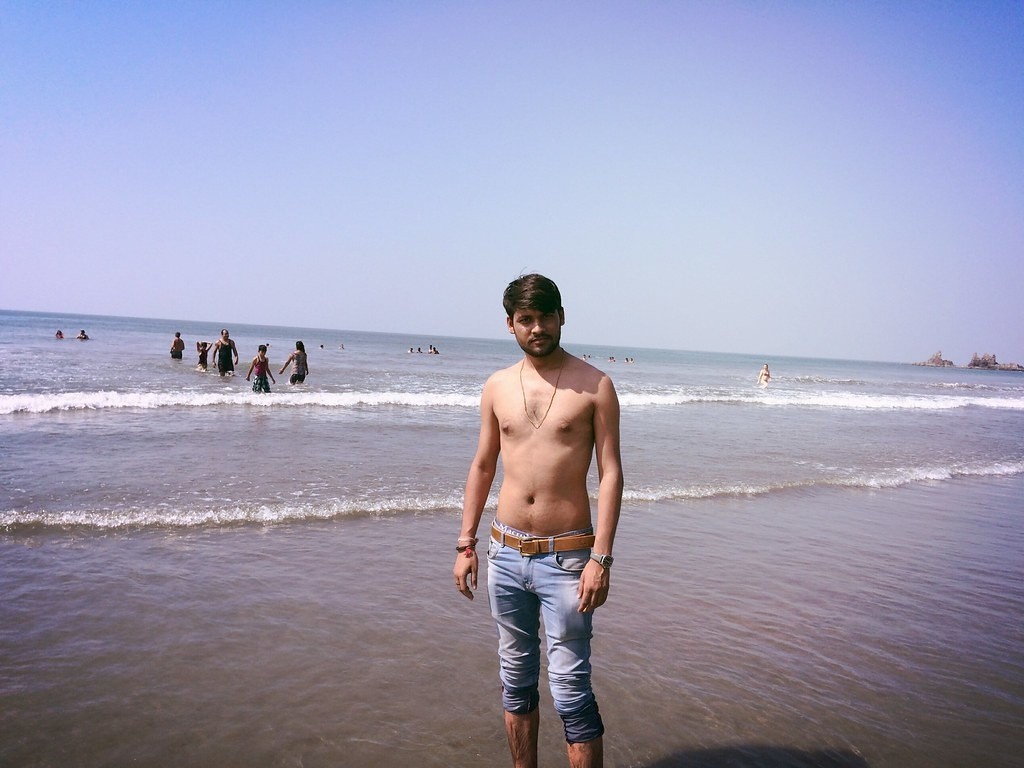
xmin=458 ymin=536 xmax=476 ymax=544
xmin=456 ymin=544 xmax=476 ymax=552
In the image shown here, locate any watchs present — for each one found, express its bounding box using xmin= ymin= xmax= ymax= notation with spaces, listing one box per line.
xmin=590 ymin=553 xmax=615 ymax=570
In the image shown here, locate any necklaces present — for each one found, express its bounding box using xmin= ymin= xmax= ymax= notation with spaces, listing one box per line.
xmin=520 ymin=347 xmax=565 ymax=425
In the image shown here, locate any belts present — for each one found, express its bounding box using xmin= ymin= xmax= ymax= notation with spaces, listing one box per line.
xmin=491 ymin=521 xmax=596 ymax=556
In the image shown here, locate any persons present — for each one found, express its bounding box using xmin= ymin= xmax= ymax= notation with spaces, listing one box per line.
xmin=266 ymin=343 xmax=270 ymax=347
xmin=757 ymin=364 xmax=771 ymax=388
xmin=625 ymin=357 xmax=634 ymax=363
xmin=608 ymin=357 xmax=615 ymax=362
xmin=320 ymin=345 xmax=324 ymax=349
xmin=246 ymin=345 xmax=276 ymax=393
xmin=56 ymin=330 xmax=64 ymax=338
xmin=428 ymin=344 xmax=440 ymax=354
xmin=170 ymin=332 xmax=184 ymax=359
xmin=212 ymin=329 xmax=238 ymax=377
xmin=408 ymin=347 xmax=413 ymax=353
xmin=279 ymin=341 xmax=309 ymax=385
xmin=452 ymin=275 xmax=624 ymax=768
xmin=416 ymin=348 xmax=423 ymax=353
xmin=77 ymin=330 xmax=89 ymax=340
xmin=197 ymin=341 xmax=213 ymax=371
xmin=338 ymin=344 xmax=344 ymax=350
xmin=582 ymin=354 xmax=590 ymax=361
